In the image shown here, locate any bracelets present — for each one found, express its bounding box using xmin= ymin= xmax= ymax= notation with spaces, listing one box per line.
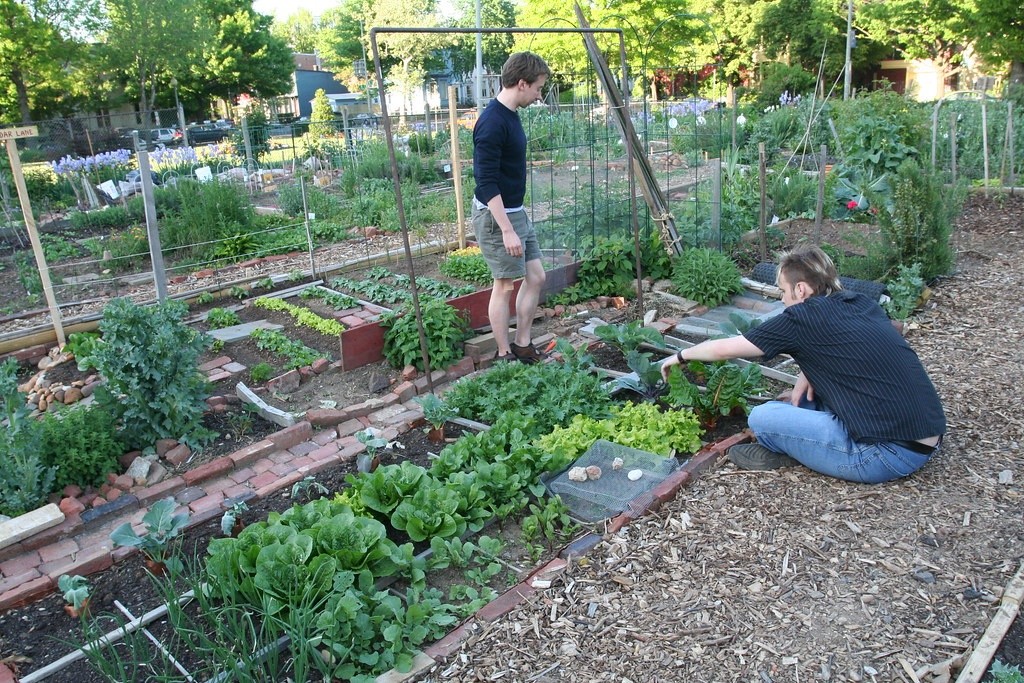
xmin=677 ymin=350 xmax=684 ymax=364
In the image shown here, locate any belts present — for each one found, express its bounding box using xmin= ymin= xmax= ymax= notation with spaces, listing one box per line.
xmin=890 ymin=440 xmax=936 ymax=455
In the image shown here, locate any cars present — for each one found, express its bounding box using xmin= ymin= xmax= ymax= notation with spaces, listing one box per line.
xmin=352 ymin=112 xmax=378 ymax=125
xmin=142 ymin=127 xmax=182 ymax=146
xmin=185 ymin=123 xmax=230 ymax=145
xmin=110 ymin=127 xmax=138 ymax=150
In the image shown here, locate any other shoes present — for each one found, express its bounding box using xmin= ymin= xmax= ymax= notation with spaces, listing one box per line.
xmin=492 ymin=351 xmax=518 ymax=366
xmin=729 ymin=443 xmax=801 ymax=471
xmin=510 ymin=339 xmax=548 ymax=364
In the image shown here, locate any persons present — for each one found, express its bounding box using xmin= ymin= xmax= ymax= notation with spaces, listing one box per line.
xmin=471 ymin=51 xmax=548 ymax=366
xmin=660 ymin=243 xmax=946 ymax=483
